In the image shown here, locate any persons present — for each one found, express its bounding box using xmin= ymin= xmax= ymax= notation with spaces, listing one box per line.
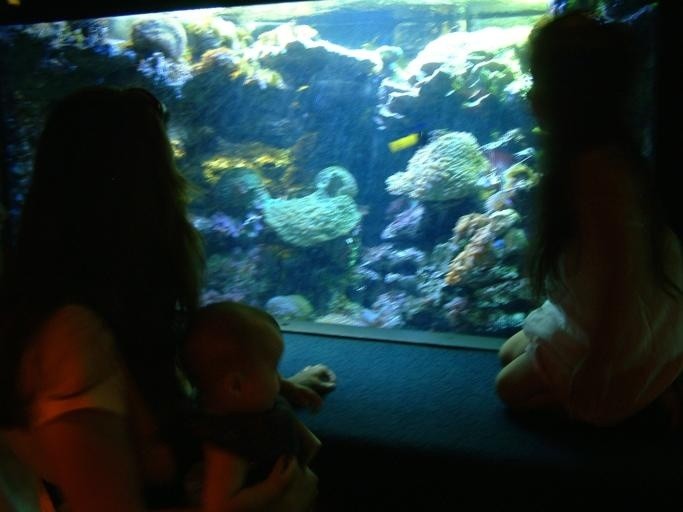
xmin=495 ymin=11 xmax=682 ymax=432
xmin=173 ymin=301 xmax=322 ymax=509
xmin=0 ymin=87 xmax=335 ymax=510
